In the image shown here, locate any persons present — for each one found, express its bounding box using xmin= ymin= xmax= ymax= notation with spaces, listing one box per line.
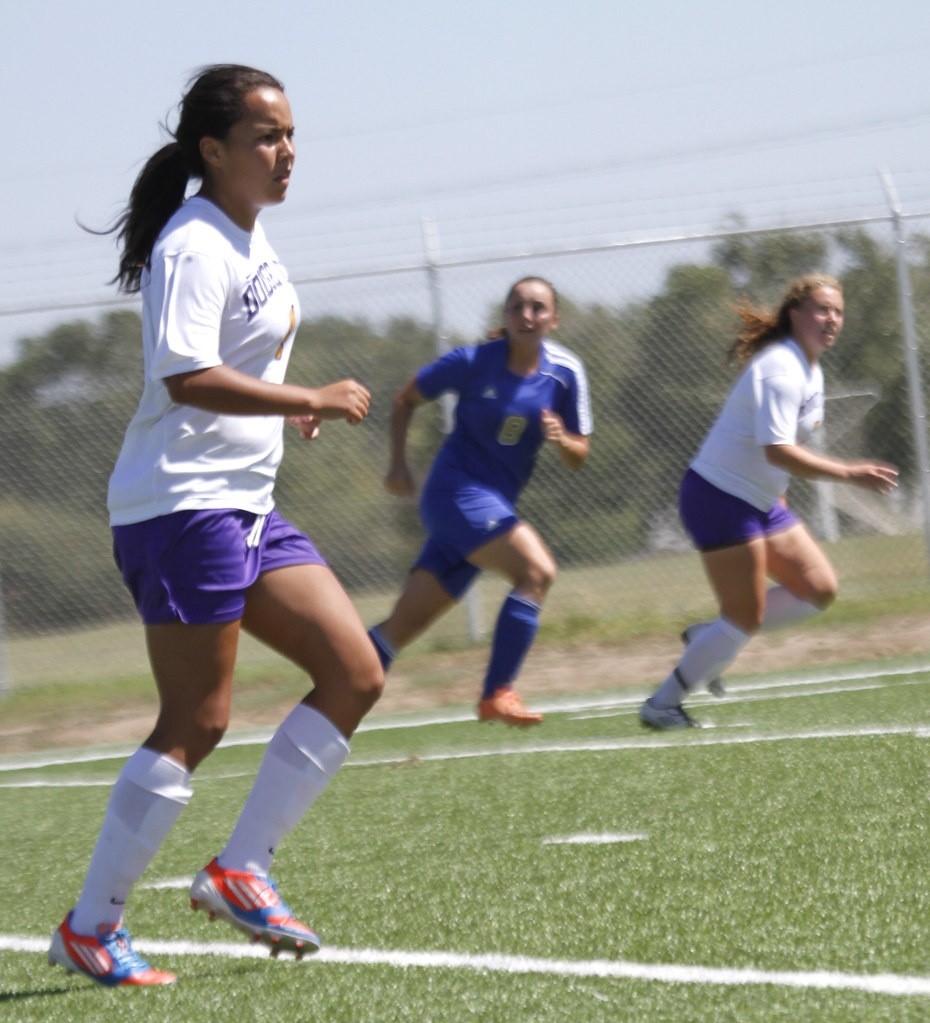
xmin=638 ymin=273 xmax=899 ymax=732
xmin=46 ymin=64 xmax=386 ymax=989
xmin=366 ymin=275 xmax=595 ymax=728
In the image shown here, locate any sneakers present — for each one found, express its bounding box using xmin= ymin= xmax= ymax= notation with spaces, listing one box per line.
xmin=47 ymin=911 xmax=177 ymax=989
xmin=190 ymin=855 xmax=320 ymax=961
xmin=639 ymin=698 xmax=703 ymax=732
xmin=681 ymin=623 xmax=726 ymax=698
xmin=478 ymin=685 xmax=542 ymax=727
